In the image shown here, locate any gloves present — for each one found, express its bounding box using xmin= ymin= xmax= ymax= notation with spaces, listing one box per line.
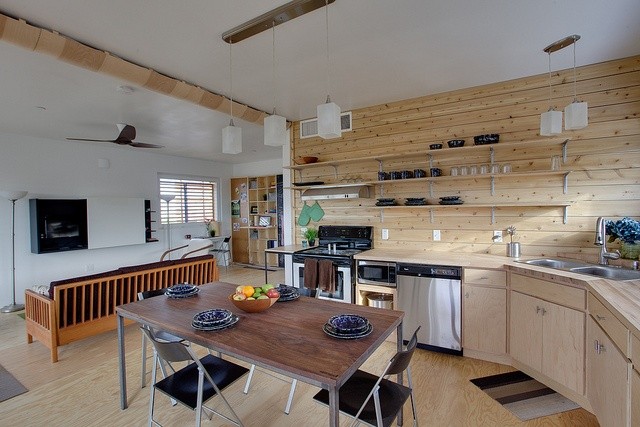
xmin=298 ymin=201 xmax=311 ymax=226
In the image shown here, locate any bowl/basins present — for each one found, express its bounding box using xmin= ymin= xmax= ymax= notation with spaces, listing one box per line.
xmin=447 ymin=140 xmax=465 ymax=148
xmin=469 ymin=165 xmax=479 ymax=175
xmin=227 ymin=292 xmax=280 ymax=313
xmin=473 ymin=134 xmax=500 ymax=145
xmin=459 ymin=166 xmax=469 ymax=176
xmin=502 ymin=163 xmax=512 ymax=173
xmin=478 ymin=165 xmax=489 ymax=175
xmin=490 ymin=163 xmax=501 ymax=174
xmin=449 ymin=167 xmax=459 ymax=176
xmin=429 ymin=144 xmax=442 ymax=149
xmin=291 ymin=157 xmax=319 ymax=164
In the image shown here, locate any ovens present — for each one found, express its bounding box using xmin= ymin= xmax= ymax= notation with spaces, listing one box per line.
xmin=292 ymin=262 xmax=354 ymax=304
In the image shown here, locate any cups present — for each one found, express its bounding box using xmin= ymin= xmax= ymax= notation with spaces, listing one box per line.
xmin=551 ymin=155 xmax=561 ymax=171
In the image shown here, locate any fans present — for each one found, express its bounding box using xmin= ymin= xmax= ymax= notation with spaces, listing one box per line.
xmin=65 ymin=124 xmax=167 ymax=149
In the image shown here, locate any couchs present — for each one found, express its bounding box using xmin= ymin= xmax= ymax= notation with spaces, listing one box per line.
xmin=25 ymin=255 xmax=220 ymax=363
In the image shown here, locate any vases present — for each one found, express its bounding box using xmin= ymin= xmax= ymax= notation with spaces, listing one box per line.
xmin=620 ymin=244 xmax=639 ymax=259
xmin=205 ymin=229 xmax=211 ymax=237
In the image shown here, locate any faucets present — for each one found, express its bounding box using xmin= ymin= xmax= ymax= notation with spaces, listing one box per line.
xmin=592 ymin=217 xmax=620 ymax=265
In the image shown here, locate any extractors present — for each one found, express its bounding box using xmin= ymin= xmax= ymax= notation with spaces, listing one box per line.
xmin=301 ymin=186 xmax=370 ymax=200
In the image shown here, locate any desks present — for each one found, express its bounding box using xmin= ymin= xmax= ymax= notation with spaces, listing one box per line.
xmin=188 ymin=235 xmax=227 ymax=254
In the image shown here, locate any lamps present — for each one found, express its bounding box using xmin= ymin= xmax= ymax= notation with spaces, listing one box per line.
xmin=564 ymin=41 xmax=588 ymax=132
xmin=540 ymin=53 xmax=563 ymax=137
xmin=316 ymin=0 xmax=344 ymax=140
xmin=160 ymin=195 xmax=176 ymax=260
xmin=221 ymin=39 xmax=243 ymax=155
xmin=263 ymin=21 xmax=287 ymax=148
xmin=0 ymin=190 xmax=29 ymax=314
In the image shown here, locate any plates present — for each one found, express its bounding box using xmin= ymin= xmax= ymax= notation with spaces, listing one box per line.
xmin=192 ymin=308 xmax=232 ymax=326
xmin=164 ymin=288 xmax=200 ymax=298
xmin=404 ymin=197 xmax=425 ymax=202
xmin=376 ymin=198 xmax=395 ymax=202
xmin=277 ymin=288 xmax=300 ymax=302
xmin=328 ymin=314 xmax=370 ymax=333
xmin=189 ymin=315 xmax=239 ymax=331
xmin=275 ymin=285 xmax=296 ymax=296
xmin=403 ymin=202 xmax=426 ymax=205
xmin=291 ymin=181 xmax=324 ymax=185
xmin=438 ymin=201 xmax=464 ymax=205
xmin=322 ymin=321 xmax=373 ymax=340
xmin=438 ymin=196 xmax=461 ymax=200
xmin=375 ymin=202 xmax=397 ymax=206
xmin=167 ymin=284 xmax=197 ymax=294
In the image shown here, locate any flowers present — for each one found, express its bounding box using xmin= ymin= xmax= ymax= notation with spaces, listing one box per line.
xmin=205 ymin=222 xmax=211 ymax=230
xmin=605 ymin=216 xmax=640 ymax=243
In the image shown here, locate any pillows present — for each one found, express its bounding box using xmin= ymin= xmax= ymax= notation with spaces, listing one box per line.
xmin=49 ymin=268 xmax=119 ymax=328
xmin=119 ymin=260 xmax=170 ymax=312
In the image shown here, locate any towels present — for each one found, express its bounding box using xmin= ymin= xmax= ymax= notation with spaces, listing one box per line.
xmin=318 ymin=259 xmax=336 ymax=293
xmin=303 ymin=258 xmax=318 ymax=291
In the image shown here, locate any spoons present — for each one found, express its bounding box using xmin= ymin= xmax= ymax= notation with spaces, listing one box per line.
xmin=506 ymin=228 xmax=513 ymax=243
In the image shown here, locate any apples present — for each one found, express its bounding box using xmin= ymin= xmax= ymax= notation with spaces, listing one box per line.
xmin=236 ymin=286 xmax=243 ymax=295
xmin=257 ymin=296 xmax=269 ymax=300
xmin=268 ymin=290 xmax=278 ymax=299
xmin=262 ymin=284 xmax=274 ymax=295
xmin=246 ymin=297 xmax=255 ymax=300
xmin=253 ymin=292 xmax=261 ymax=298
xmin=234 ymin=295 xmax=246 ymax=300
xmin=255 ymin=287 xmax=261 ymax=292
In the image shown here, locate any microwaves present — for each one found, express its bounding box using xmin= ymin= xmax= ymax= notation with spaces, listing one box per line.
xmin=357 ymin=260 xmax=397 ymax=287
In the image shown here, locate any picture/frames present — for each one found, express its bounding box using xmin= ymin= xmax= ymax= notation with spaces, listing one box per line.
xmin=259 ymin=216 xmax=271 ymax=226
xmin=251 ymin=206 xmax=257 ymax=213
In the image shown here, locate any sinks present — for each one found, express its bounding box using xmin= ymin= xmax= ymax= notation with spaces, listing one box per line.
xmin=527 ymin=259 xmax=587 ymax=270
xmin=572 ymin=268 xmax=640 ymax=281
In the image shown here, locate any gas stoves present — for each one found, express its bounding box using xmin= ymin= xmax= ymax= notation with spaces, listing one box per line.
xmin=293 ymin=246 xmax=372 ymax=260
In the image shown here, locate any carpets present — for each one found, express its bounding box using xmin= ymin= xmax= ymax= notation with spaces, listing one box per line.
xmin=17 ymin=313 xmax=25 ymax=320
xmin=470 ymin=370 xmax=581 ymax=422
xmin=0 ymin=363 xmax=29 ymax=402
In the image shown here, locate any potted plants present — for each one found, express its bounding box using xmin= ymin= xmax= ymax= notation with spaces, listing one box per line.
xmin=304 ymin=228 xmax=318 ymax=246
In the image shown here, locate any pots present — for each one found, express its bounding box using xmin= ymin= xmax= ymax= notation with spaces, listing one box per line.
xmin=365 ymin=294 xmax=393 ymax=310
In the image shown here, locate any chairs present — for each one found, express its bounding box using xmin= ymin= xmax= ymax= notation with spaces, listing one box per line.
xmin=141 ymin=326 xmax=250 ymax=427
xmin=312 ymin=325 xmax=422 ymax=427
xmin=138 ymin=288 xmax=213 ymax=407
xmin=243 ymin=282 xmax=321 ymax=415
xmin=208 ymin=236 xmax=232 ymax=272
xmin=160 ymin=239 xmax=214 ymax=263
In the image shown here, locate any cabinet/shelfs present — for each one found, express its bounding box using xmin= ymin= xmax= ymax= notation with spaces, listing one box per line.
xmin=462 ymin=269 xmax=507 ymax=356
xmin=230 ymin=176 xmax=278 ymax=267
xmin=509 ymin=273 xmax=586 ymax=396
xmin=282 ymin=138 xmax=570 ymax=226
xmin=629 ymin=322 xmax=640 ymax=427
xmin=588 ymin=292 xmax=630 ymax=427
